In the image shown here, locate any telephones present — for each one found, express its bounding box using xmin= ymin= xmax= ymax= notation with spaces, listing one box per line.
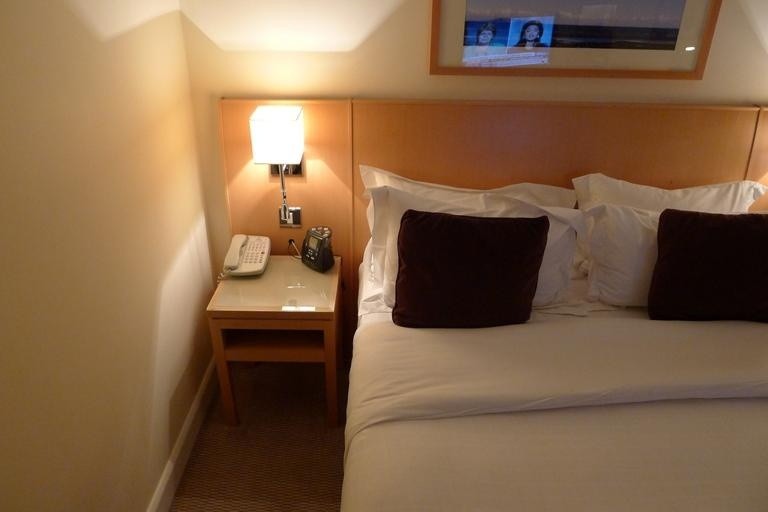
xmin=223 ymin=234 xmax=271 ymax=277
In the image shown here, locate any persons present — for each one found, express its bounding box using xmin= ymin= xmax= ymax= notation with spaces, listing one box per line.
xmin=511 ymin=19 xmax=548 ymax=53
xmin=464 ymin=22 xmax=505 ymax=58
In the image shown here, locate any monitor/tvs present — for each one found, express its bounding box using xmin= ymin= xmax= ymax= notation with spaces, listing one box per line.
xmin=462 ymin=16 xmax=553 ymax=68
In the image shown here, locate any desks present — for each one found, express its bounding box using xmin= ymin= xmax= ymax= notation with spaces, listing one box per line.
xmin=207 ymin=254 xmax=340 ymax=428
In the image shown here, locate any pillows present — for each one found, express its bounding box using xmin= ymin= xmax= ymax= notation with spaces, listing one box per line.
xmin=575 ymin=197 xmax=661 ymax=308
xmin=359 ymin=184 xmax=588 ymax=316
xmin=647 ymin=208 xmax=766 ymax=324
xmin=571 ymin=173 xmax=768 ymax=213
xmin=392 ymin=208 xmax=550 ymax=329
xmin=358 ymin=165 xmax=576 ymax=233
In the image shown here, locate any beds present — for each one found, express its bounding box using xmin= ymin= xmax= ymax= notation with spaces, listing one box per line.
xmin=344 ymin=97 xmax=767 ymax=512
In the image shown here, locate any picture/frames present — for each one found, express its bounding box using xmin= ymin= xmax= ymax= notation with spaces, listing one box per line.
xmin=429 ymin=0 xmax=723 ymax=82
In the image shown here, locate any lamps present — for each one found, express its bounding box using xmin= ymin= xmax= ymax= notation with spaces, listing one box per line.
xmin=249 ymin=104 xmax=308 ymax=229
